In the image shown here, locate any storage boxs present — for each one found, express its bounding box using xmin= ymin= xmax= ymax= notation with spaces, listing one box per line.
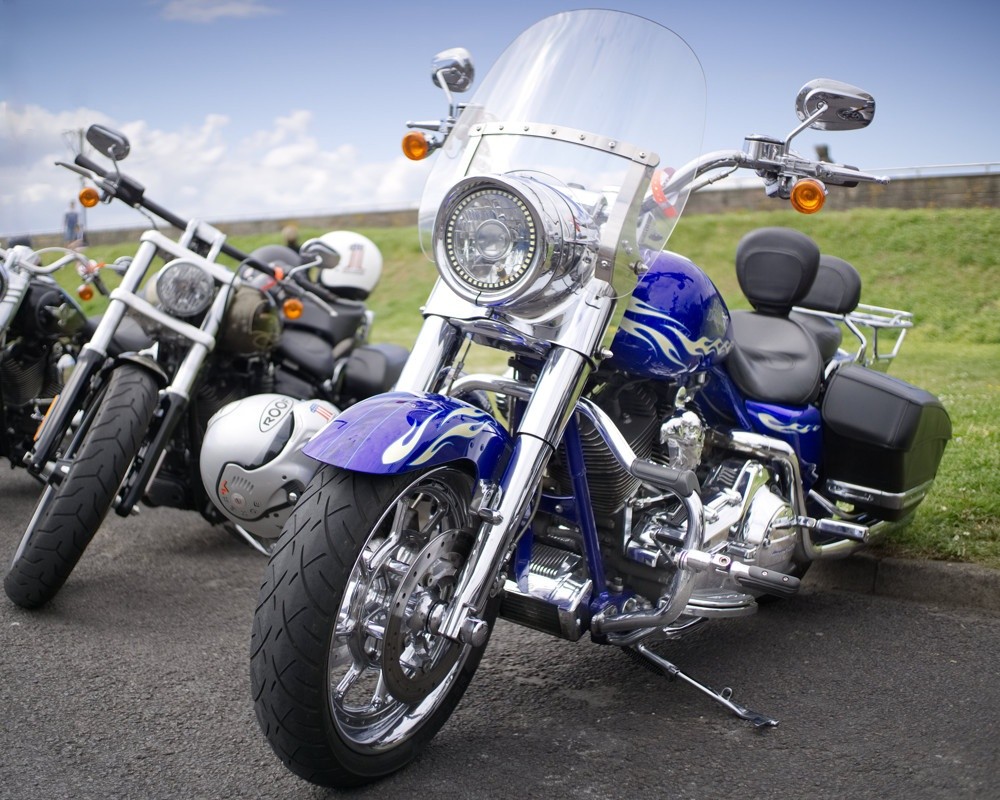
xmin=821 ymin=365 xmax=952 ymax=521
xmin=340 ymin=343 xmax=409 ymax=409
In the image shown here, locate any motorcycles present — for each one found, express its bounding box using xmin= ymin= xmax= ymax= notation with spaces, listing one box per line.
xmin=243 ymin=2 xmax=955 ymax=793
xmin=0 ymin=242 xmax=111 ymax=482
xmin=4 ymin=123 xmax=416 ymax=611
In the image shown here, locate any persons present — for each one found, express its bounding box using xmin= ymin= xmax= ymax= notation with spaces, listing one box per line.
xmin=63 ymin=201 xmax=80 ymax=247
